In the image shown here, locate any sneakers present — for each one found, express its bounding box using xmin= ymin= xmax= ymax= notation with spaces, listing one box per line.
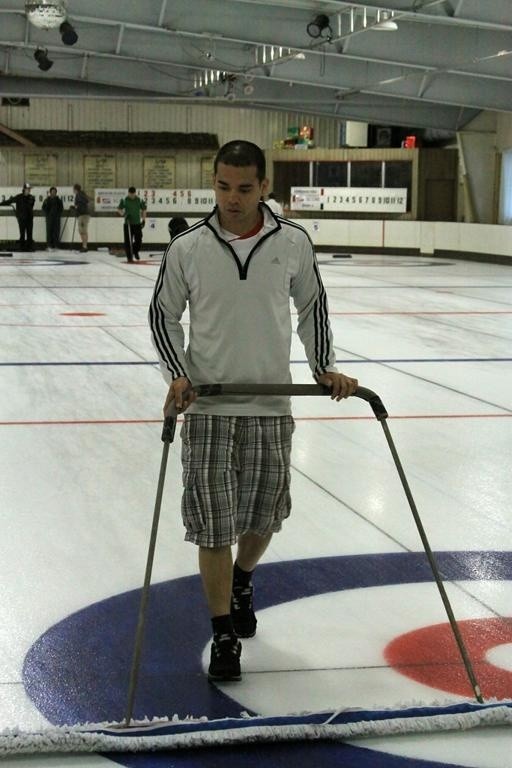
xmin=231 ymin=578 xmax=257 ymax=639
xmin=205 ymin=635 xmax=244 ymax=683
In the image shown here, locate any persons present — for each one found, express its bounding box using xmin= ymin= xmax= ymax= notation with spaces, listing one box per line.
xmin=0 ymin=181 xmax=37 ymax=244
xmin=149 ymin=138 xmax=359 ymax=681
xmin=116 ymin=186 xmax=147 ymax=264
xmin=69 ymin=184 xmax=92 ymax=253
xmin=263 ymin=191 xmax=284 ymax=219
xmin=42 ymin=186 xmax=66 ymax=250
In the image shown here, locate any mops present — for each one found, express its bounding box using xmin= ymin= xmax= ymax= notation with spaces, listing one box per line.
xmin=120 ymin=214 xmax=147 ymax=263
xmin=0 ymin=375 xmax=511 ymax=755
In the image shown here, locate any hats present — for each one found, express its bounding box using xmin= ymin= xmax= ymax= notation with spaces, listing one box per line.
xmin=23 ymin=182 xmax=33 ymax=189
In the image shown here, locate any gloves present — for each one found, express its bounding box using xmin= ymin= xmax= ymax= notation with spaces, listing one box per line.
xmin=139 ymin=220 xmax=145 ymax=229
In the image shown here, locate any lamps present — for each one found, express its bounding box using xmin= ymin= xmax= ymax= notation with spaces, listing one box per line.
xmin=306 ymin=15 xmax=334 ymax=44
xmin=220 ymin=71 xmax=253 ymax=102
xmin=372 ymin=11 xmax=399 ymax=32
xmin=24 ymin=1 xmax=67 ymax=30
xmin=33 ymin=43 xmax=53 ymax=72
xmin=60 ymin=22 xmax=78 ymax=45
